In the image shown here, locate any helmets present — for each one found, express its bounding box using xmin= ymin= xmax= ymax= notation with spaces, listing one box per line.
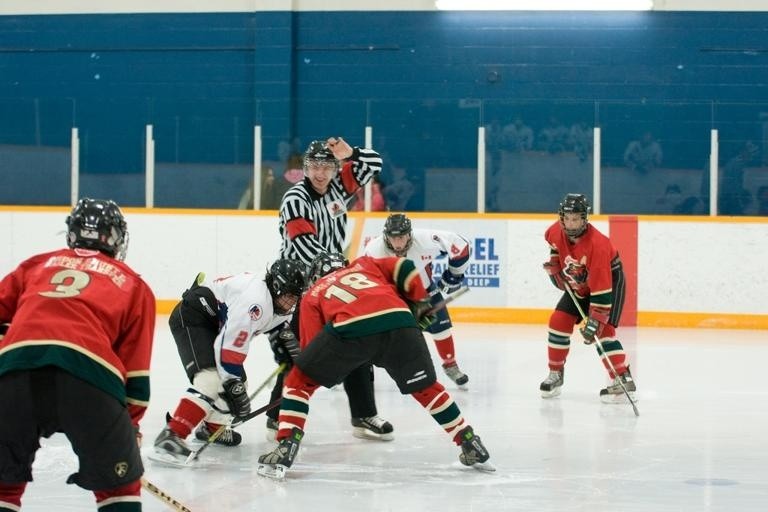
xmin=266 ymin=259 xmax=305 ymax=317
xmin=382 ymin=214 xmax=412 ymax=256
xmin=304 ymin=139 xmax=337 ymax=180
xmin=65 ymin=197 xmax=128 ymax=260
xmin=558 ymin=193 xmax=589 ymax=239
xmin=308 ymin=252 xmax=348 ymax=285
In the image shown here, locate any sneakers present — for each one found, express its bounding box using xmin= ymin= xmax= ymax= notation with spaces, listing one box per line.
xmin=351 ymin=415 xmax=394 ymax=433
xmin=443 ymin=361 xmax=469 ymax=385
xmin=540 ymin=369 xmax=563 ymax=391
xmin=459 ymin=429 xmax=490 ymax=465
xmin=154 ymin=425 xmax=198 ymax=461
xmin=257 ymin=427 xmax=304 ymax=467
xmin=599 ymin=372 xmax=636 ymax=395
xmin=196 ymin=421 xmax=241 ymax=446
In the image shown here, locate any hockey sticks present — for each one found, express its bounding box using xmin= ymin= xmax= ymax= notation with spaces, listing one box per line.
xmin=204 ymin=286 xmax=469 ymax=425
xmin=563 ymin=280 xmax=639 ymax=416
xmin=148 ymin=361 xmax=285 ymax=467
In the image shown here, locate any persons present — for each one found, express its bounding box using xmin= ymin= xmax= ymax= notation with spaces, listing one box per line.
xmin=258 ymin=252 xmax=496 ymax=478
xmin=540 ymin=191 xmax=639 ymax=391
xmin=0 ymin=195 xmax=157 ymax=512
xmin=484 ymin=113 xmax=768 ymax=214
xmin=238 ymin=151 xmax=386 ymax=212
xmin=147 ymin=258 xmax=305 ymax=466
xmin=266 ymin=136 xmax=394 ymax=441
xmin=352 ymin=214 xmax=470 ymax=390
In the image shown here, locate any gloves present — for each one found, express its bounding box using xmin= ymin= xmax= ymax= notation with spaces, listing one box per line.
xmin=543 ymin=263 xmax=564 ymax=290
xmin=438 ymin=270 xmax=464 ymax=295
xmin=581 ymin=311 xmax=608 ymax=343
xmin=268 ymin=327 xmax=301 ymax=362
xmin=221 ymin=379 xmax=251 ymax=427
xmin=415 ymin=300 xmax=438 ymax=330
